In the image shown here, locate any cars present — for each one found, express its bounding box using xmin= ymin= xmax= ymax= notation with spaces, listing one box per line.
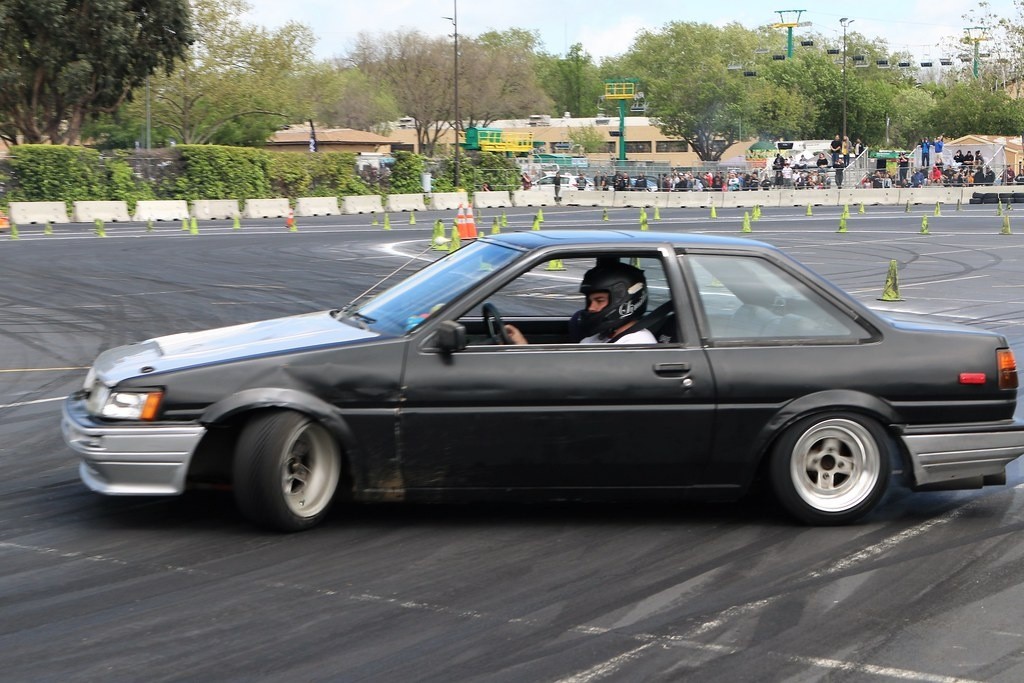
xmin=593 ymin=174 xmax=710 ymax=191
xmin=518 ymin=173 xmax=595 ymax=190
xmin=60 ymin=226 xmax=1024 ymax=528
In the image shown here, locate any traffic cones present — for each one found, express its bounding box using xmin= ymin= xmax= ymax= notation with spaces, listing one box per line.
xmin=456 ymin=204 xmax=472 ymax=240
xmin=370 ymin=211 xmax=380 ymax=226
xmin=640 ymin=212 xmax=649 ymax=231
xmin=627 ymin=258 xmax=641 ymax=269
xmin=382 ymin=213 xmax=392 ymax=230
xmin=834 ymin=212 xmax=851 ymax=233
xmin=543 ymin=260 xmax=567 ymax=271
xmin=476 ymin=230 xmax=491 ymax=271
xmin=408 ymin=211 xmax=417 ymax=225
xmin=917 ymin=214 xmax=931 ymax=236
xmin=427 ymin=219 xmax=450 ymax=251
xmin=475 ymin=207 xmax=546 ymax=233
xmin=445 ymin=217 xmax=461 ymax=255
xmin=465 ymin=203 xmax=479 ymax=239
xmin=876 ymin=259 xmax=907 ymax=302
xmin=7 ymin=207 xmax=299 ymax=239
xmin=739 ymin=212 xmax=752 ymax=233
xmin=998 ymin=214 xmax=1014 ymax=235
xmin=602 ymin=197 xmax=1014 ymax=221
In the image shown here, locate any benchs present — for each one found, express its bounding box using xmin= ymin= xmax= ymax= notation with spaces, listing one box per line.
xmin=734 ymin=305 xmax=822 ymax=336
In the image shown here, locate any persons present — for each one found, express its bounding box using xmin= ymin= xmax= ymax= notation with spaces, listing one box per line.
xmin=520 ymin=171 xmax=532 ymax=190
xmin=576 ymin=173 xmax=586 ymax=190
xmin=853 ymin=138 xmax=864 ymax=158
xmin=773 ymin=153 xmax=831 ymax=189
xmin=1015 ymin=169 xmax=1024 ymax=185
xmin=635 ymin=172 xmax=647 ymax=191
xmin=833 ymin=157 xmax=845 ymax=189
xmin=553 ymin=173 xmax=560 ymax=197
xmin=863 ymin=150 xmax=996 ymax=188
xmin=657 ymin=169 xmax=761 ymax=192
xmin=921 ymin=137 xmax=930 ymax=167
xmin=761 ymin=177 xmax=772 ymax=190
xmin=593 ymin=171 xmax=632 ymax=191
xmin=504 ymin=262 xmax=658 ymax=345
xmin=482 ymin=183 xmax=492 ymax=192
xmin=830 ymin=134 xmax=842 ymax=167
xmin=1003 ymin=164 xmax=1015 ymax=185
xmin=933 ymin=137 xmax=943 ymax=159
xmin=842 ymin=135 xmax=852 ymax=167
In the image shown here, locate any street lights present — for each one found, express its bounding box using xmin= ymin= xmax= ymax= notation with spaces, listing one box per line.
xmin=839 ymin=17 xmax=856 ymax=135
xmin=441 ymin=0 xmax=460 ymax=189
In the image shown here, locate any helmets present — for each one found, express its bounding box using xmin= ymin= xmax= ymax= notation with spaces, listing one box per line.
xmin=573 ymin=262 xmax=648 ymax=337
